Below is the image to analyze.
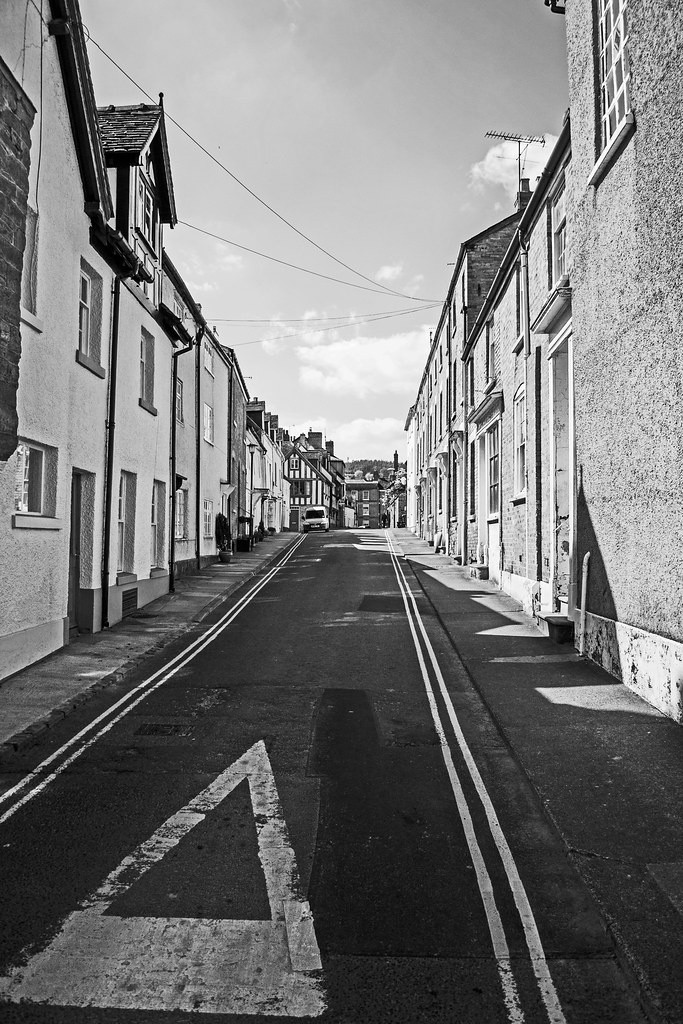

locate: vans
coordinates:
[301,505,330,533]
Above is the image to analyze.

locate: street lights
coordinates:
[247,442,258,539]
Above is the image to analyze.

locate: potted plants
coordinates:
[215,513,234,563]
[268,527,276,536]
